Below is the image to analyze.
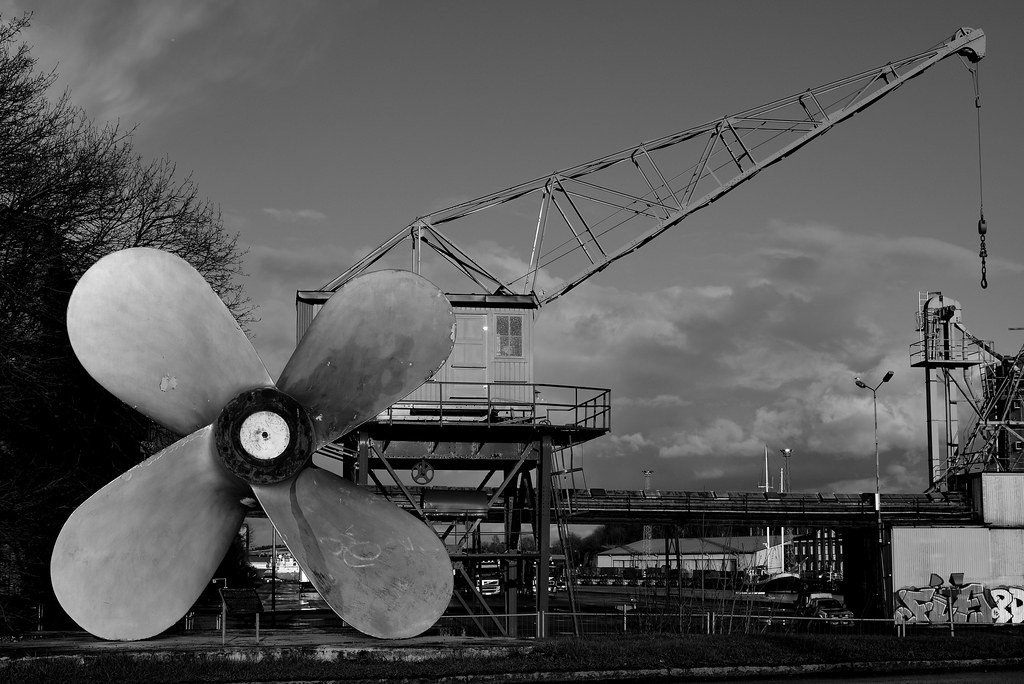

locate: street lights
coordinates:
[780,448,795,574]
[640,468,654,582]
[854,371,894,628]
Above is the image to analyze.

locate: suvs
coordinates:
[809,597,854,634]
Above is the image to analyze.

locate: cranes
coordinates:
[294,27,988,638]
